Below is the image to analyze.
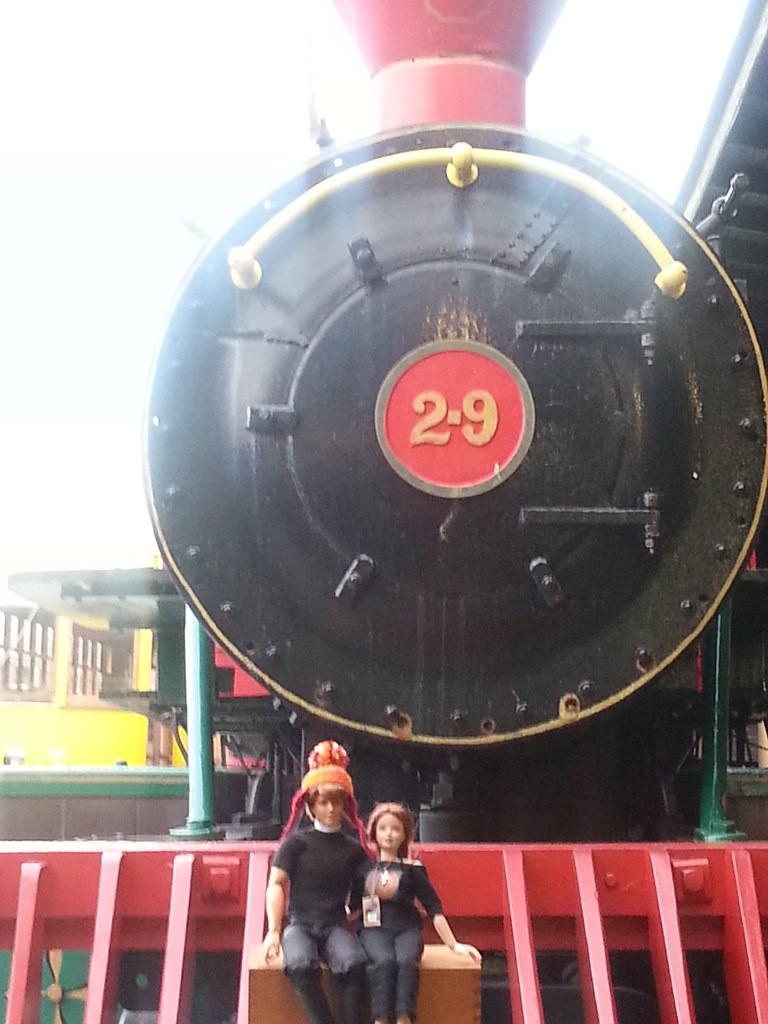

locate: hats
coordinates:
[280,740,376,860]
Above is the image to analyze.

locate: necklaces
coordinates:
[380,861,392,886]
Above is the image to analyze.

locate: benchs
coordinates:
[248,943,482,1024]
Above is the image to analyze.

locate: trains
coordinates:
[140,0,767,987]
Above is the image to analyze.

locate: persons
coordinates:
[347,801,482,1024]
[262,740,371,1024]
